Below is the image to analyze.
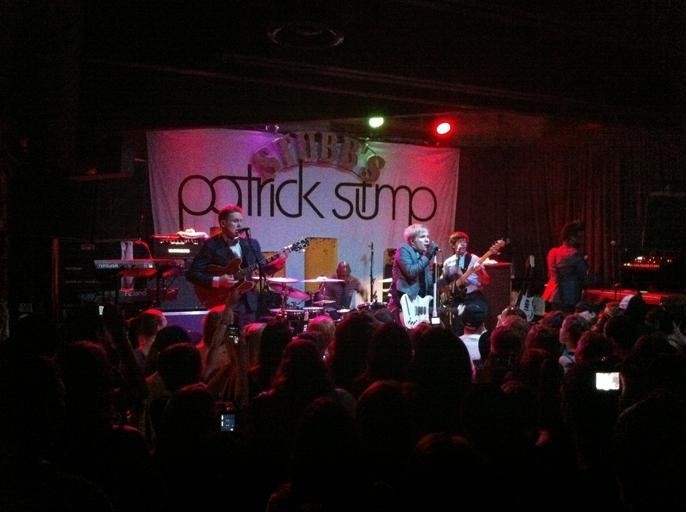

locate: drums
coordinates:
[313,300,338,322]
[302,307,325,330]
[270,308,305,334]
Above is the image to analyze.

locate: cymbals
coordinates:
[303,276,346,283]
[268,285,310,304]
[253,275,301,283]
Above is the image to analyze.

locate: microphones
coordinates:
[238,227,249,233]
[457,244,461,251]
[432,240,441,252]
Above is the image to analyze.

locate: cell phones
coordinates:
[593,369,622,392]
[216,408,238,433]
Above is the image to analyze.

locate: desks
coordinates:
[162,311,209,339]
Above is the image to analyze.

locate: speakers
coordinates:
[483,262,512,331]
[156,262,207,310]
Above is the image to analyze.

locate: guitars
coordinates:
[396,293,466,333]
[192,238,309,313]
[439,239,505,305]
[515,254,535,323]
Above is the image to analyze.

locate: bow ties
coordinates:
[227,239,238,247]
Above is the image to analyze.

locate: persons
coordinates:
[438,231,492,319]
[324,261,367,320]
[0,294,686,511]
[389,223,443,312]
[540,222,611,313]
[185,204,266,329]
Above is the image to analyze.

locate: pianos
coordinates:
[160,240,204,258]
[94,259,188,277]
